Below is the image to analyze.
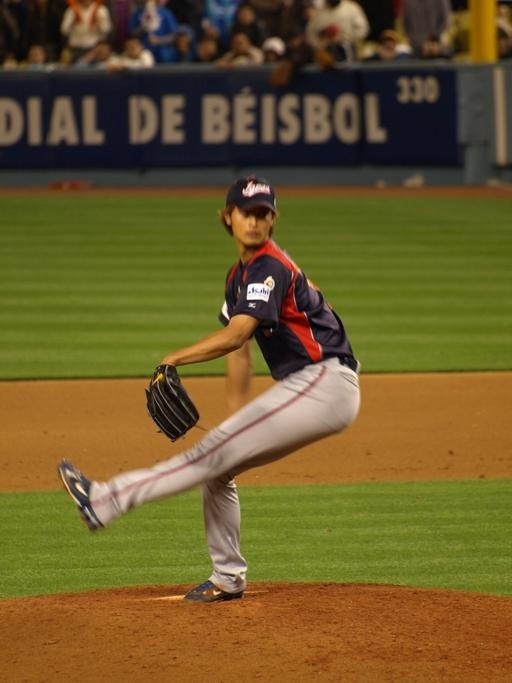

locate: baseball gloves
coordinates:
[147,363,199,441]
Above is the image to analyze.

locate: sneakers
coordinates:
[58,458,101,530]
[185,581,244,603]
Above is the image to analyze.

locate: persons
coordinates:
[56,176,361,601]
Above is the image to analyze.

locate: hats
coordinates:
[318,25,338,40]
[226,177,277,217]
[262,37,285,55]
